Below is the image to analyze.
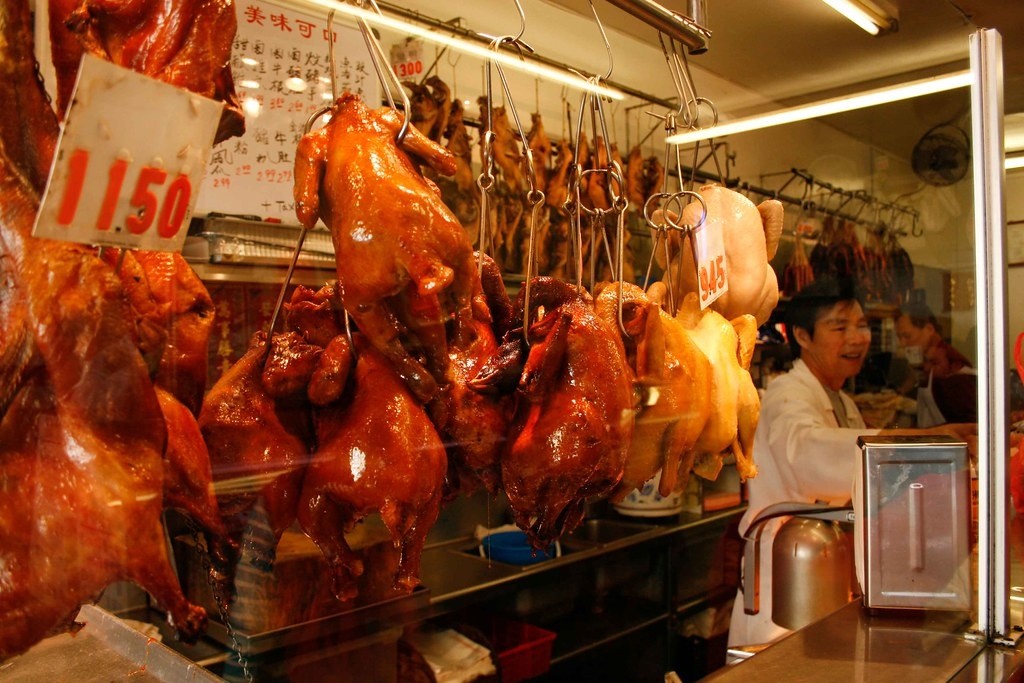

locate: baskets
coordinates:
[464,612,558,683]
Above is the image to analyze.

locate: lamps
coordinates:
[664,69,972,145]
[823,0,898,37]
[289,0,626,103]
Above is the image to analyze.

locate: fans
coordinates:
[910,122,970,187]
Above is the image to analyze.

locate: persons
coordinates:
[924,334,972,381]
[726,280,977,664]
[893,301,978,428]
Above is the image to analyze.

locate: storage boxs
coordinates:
[674,631,729,682]
[398,616,557,683]
[176,532,398,632]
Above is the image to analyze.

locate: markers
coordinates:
[208,211,262,223]
[262,216,300,227]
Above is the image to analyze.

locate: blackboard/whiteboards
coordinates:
[190,0,380,233]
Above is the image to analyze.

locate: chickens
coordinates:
[0,0,916,657]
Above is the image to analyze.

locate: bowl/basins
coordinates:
[481,531,555,565]
